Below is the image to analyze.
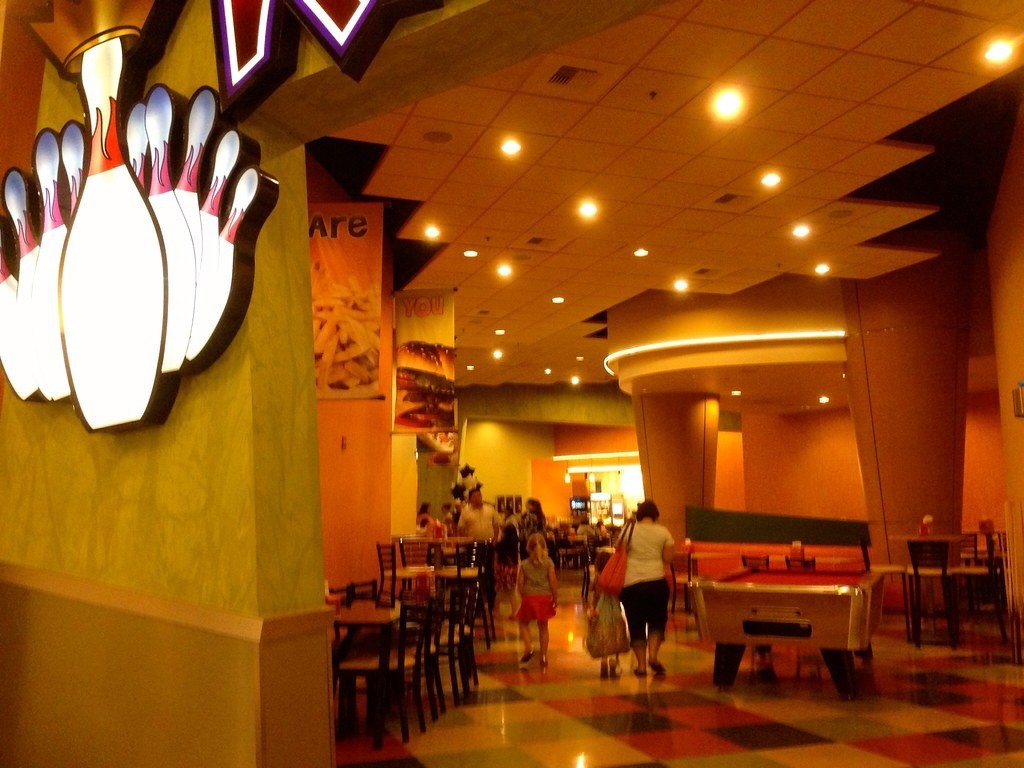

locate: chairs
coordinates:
[904,538,961,647]
[328,536,499,747]
[670,551,701,617]
[969,516,1006,644]
[555,529,610,602]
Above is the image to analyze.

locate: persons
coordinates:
[615,499,674,678]
[516,533,558,667]
[586,551,630,679]
[416,485,614,616]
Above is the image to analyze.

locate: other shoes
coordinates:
[521,646,534,663]
[540,652,548,666]
[610,659,621,679]
[600,661,608,678]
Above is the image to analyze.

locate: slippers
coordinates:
[634,668,647,677]
[649,659,664,672]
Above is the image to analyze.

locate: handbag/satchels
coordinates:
[599,519,635,594]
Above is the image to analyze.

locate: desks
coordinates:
[697,566,883,702]
[330,607,400,725]
[386,565,484,582]
[892,534,976,635]
